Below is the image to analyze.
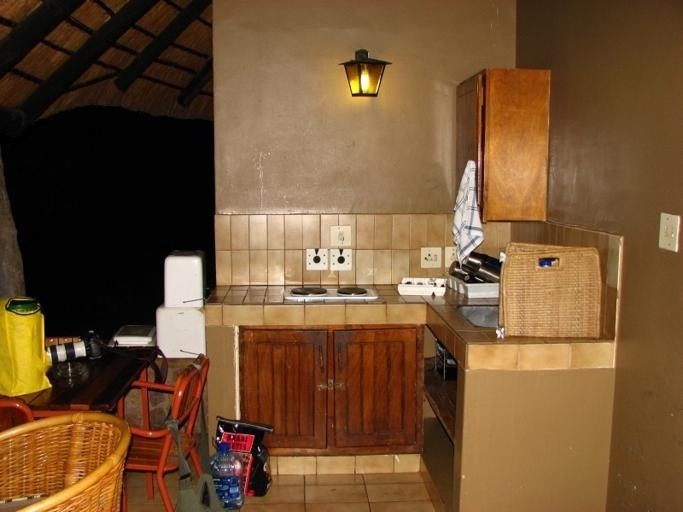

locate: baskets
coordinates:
[497,239,599,338]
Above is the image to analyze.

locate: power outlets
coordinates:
[420,247,441,269]
[445,246,459,269]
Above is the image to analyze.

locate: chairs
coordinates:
[116,353,210,512]
[0,397,36,434]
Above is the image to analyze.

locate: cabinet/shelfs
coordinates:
[420,325,614,512]
[455,67,552,223]
[237,323,425,456]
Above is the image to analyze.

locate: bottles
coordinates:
[211,440,242,508]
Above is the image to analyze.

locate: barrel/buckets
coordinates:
[209,443,244,509]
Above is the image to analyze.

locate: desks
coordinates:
[9,346,161,503]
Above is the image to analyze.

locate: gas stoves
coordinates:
[282,282,374,302]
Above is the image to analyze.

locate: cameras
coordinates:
[46,338,104,361]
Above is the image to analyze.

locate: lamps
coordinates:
[338,48,392,97]
